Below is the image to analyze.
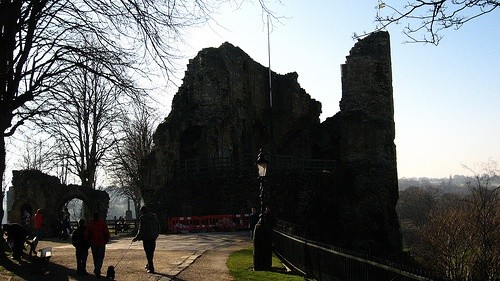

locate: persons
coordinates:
[132,205,160,273]
[249,206,259,239]
[3,224,39,260]
[59,215,73,241]
[71,213,110,277]
[34,209,42,230]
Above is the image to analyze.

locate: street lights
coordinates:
[256,147,269,224]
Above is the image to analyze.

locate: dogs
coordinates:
[105,265,116,281]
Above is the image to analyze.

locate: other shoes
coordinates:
[145,264,154,273]
[76,270,86,277]
